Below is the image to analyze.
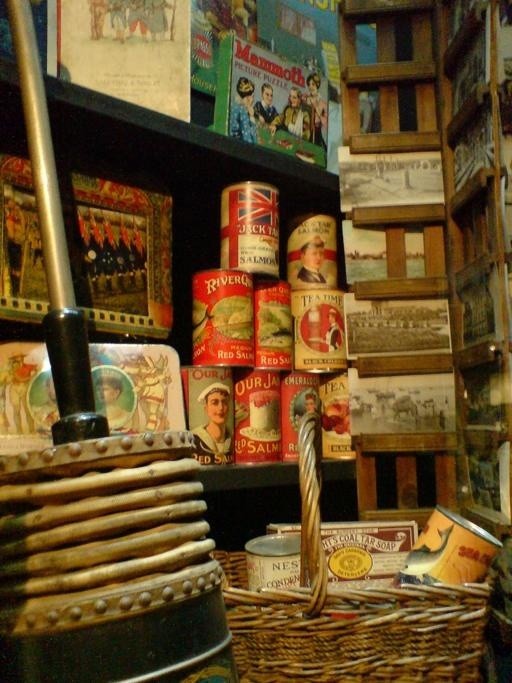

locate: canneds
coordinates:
[234,368,281,466]
[180,365,234,467]
[282,370,322,463]
[191,270,254,367]
[393,504,503,589]
[221,180,280,279]
[254,280,294,370]
[286,212,338,289]
[245,533,302,591]
[322,372,355,460]
[291,289,347,373]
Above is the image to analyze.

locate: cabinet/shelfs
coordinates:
[0,1,357,503]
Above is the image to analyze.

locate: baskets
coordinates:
[209,410,492,682]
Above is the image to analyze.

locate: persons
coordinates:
[32,374,59,424]
[303,392,319,426]
[296,234,327,284]
[90,0,177,46]
[94,368,129,428]
[292,405,307,426]
[5,188,148,299]
[308,308,343,355]
[193,383,233,454]
[204,0,328,146]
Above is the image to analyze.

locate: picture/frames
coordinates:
[0,150,176,339]
